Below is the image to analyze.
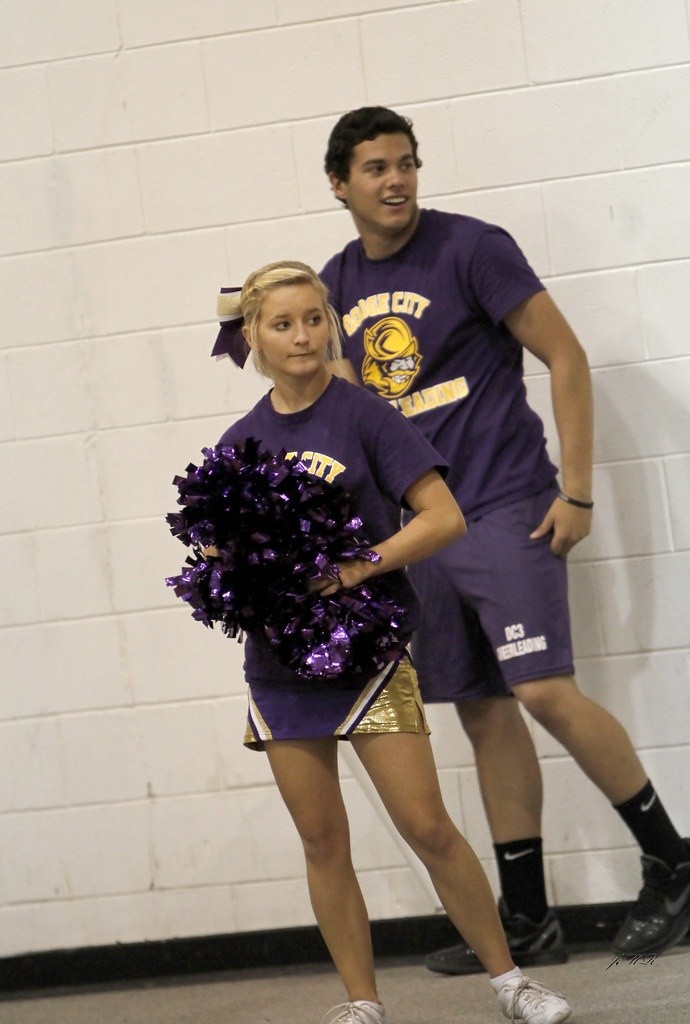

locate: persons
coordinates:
[186,261,576,1024]
[309,105,690,972]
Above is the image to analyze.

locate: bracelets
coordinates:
[557,487,595,512]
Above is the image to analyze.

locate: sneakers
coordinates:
[490,979,573,1024]
[610,835,689,962]
[422,896,571,975]
[327,999,387,1024]
